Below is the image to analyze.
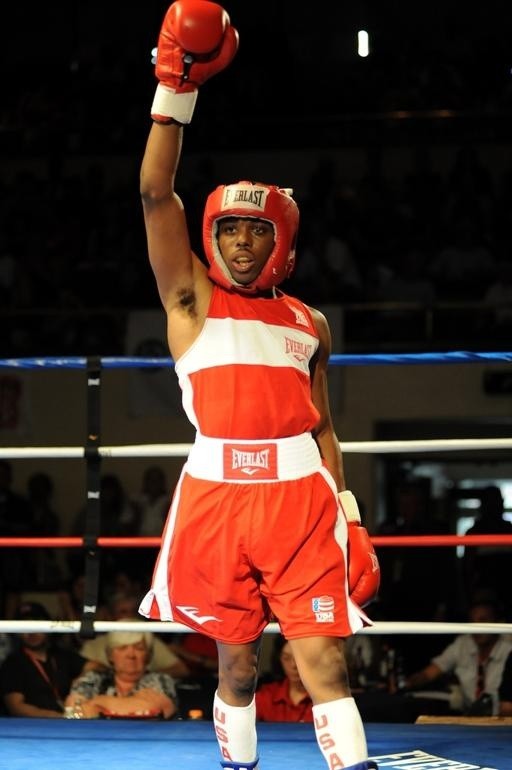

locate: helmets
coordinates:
[201,179,301,296]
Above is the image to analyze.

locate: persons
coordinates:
[137,1,381,770]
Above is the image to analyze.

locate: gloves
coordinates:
[148,0,240,127]
[336,489,381,609]
